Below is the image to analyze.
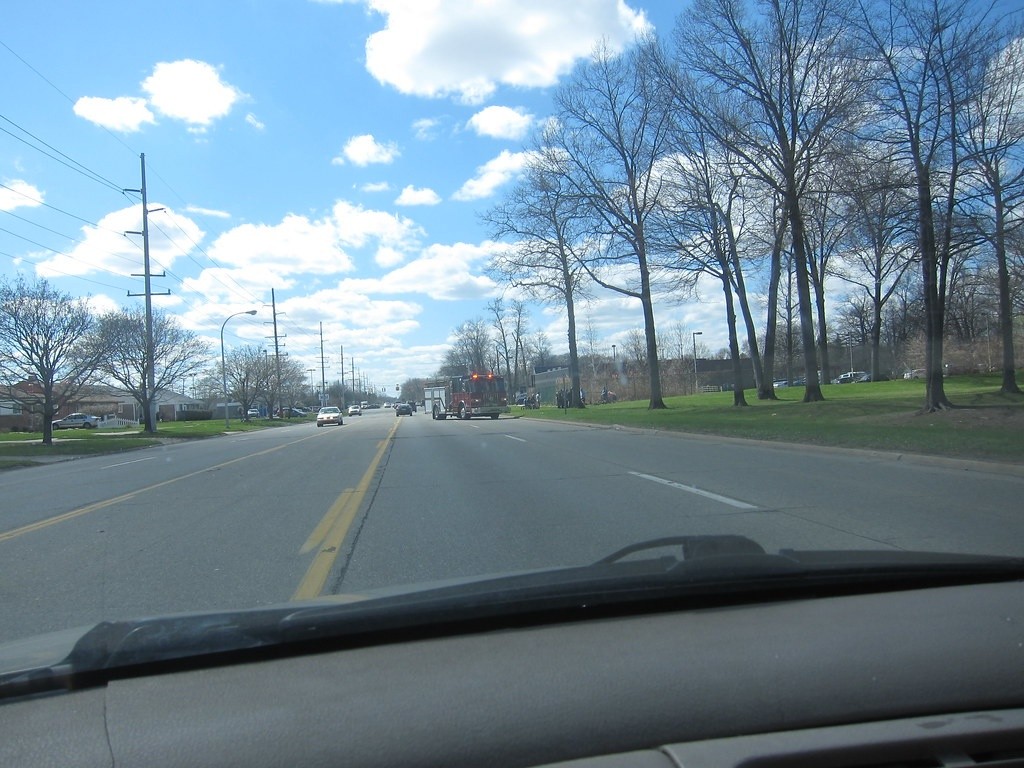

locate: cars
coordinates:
[51,413,101,430]
[396,404,412,417]
[276,407,307,418]
[772,370,888,389]
[247,408,260,418]
[904,368,928,380]
[353,399,425,413]
[317,407,344,427]
[294,405,323,415]
[348,405,362,417]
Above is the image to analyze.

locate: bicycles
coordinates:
[597,393,617,405]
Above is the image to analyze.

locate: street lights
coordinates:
[692,331,703,393]
[981,312,992,373]
[221,309,259,429]
[262,349,269,397]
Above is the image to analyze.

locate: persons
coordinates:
[530,394,536,405]
[601,386,608,404]
[567,386,573,407]
[527,397,530,403]
[580,387,585,404]
[535,392,541,409]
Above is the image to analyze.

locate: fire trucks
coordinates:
[424,373,510,420]
